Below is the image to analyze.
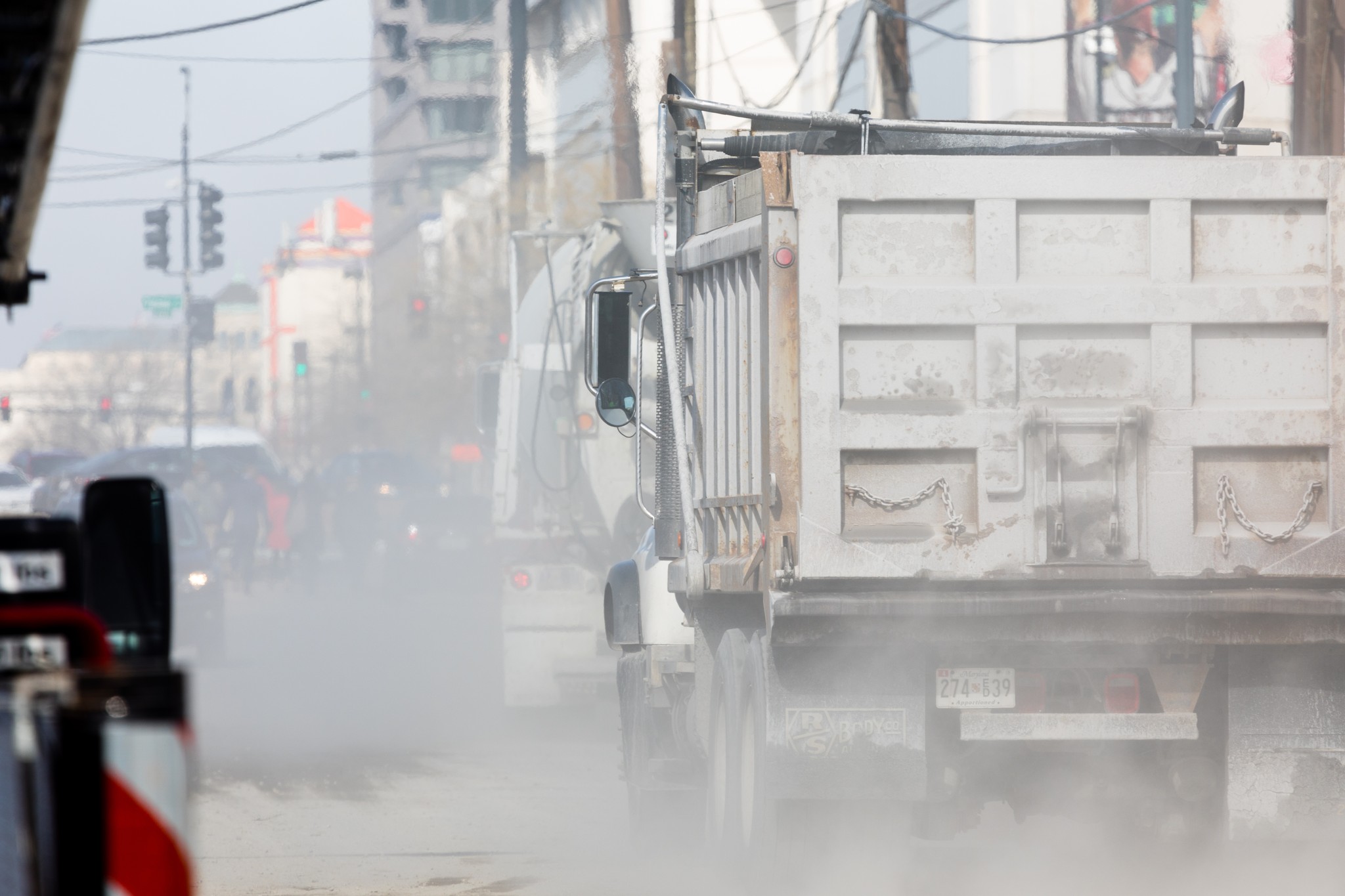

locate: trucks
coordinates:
[584,75,1345,875]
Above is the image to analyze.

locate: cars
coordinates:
[0,423,457,635]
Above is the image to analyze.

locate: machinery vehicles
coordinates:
[480,218,659,728]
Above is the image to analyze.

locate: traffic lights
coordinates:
[360,389,369,399]
[99,395,111,421]
[1,395,10,420]
[198,182,223,270]
[498,331,509,345]
[143,208,168,269]
[412,294,429,347]
[293,340,312,380]
